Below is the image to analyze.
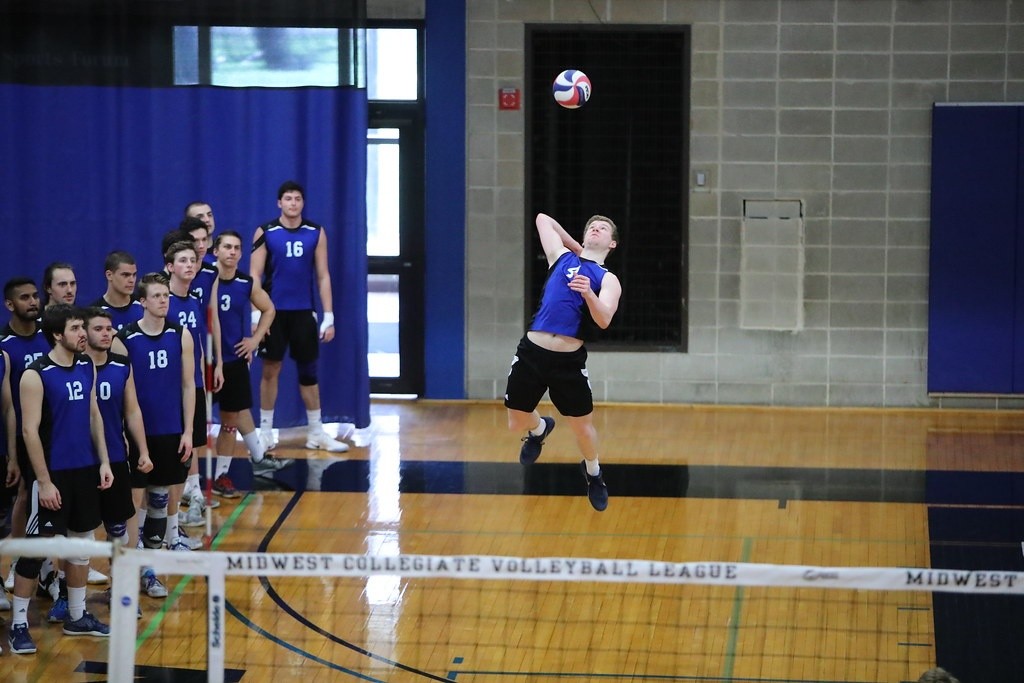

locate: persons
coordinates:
[0,199,282,622]
[6,296,113,654]
[117,273,200,597]
[248,182,350,452]
[504,213,622,512]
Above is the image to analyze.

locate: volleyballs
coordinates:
[553,68,593,111]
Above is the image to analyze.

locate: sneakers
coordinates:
[128,485,223,601]
[62,610,110,636]
[251,456,293,476]
[247,430,277,456]
[36,571,60,602]
[87,567,108,584]
[0,584,10,608]
[519,416,556,465]
[48,599,88,622]
[304,432,346,452]
[211,473,240,499]
[5,563,15,588]
[579,459,609,512]
[8,622,36,653]
